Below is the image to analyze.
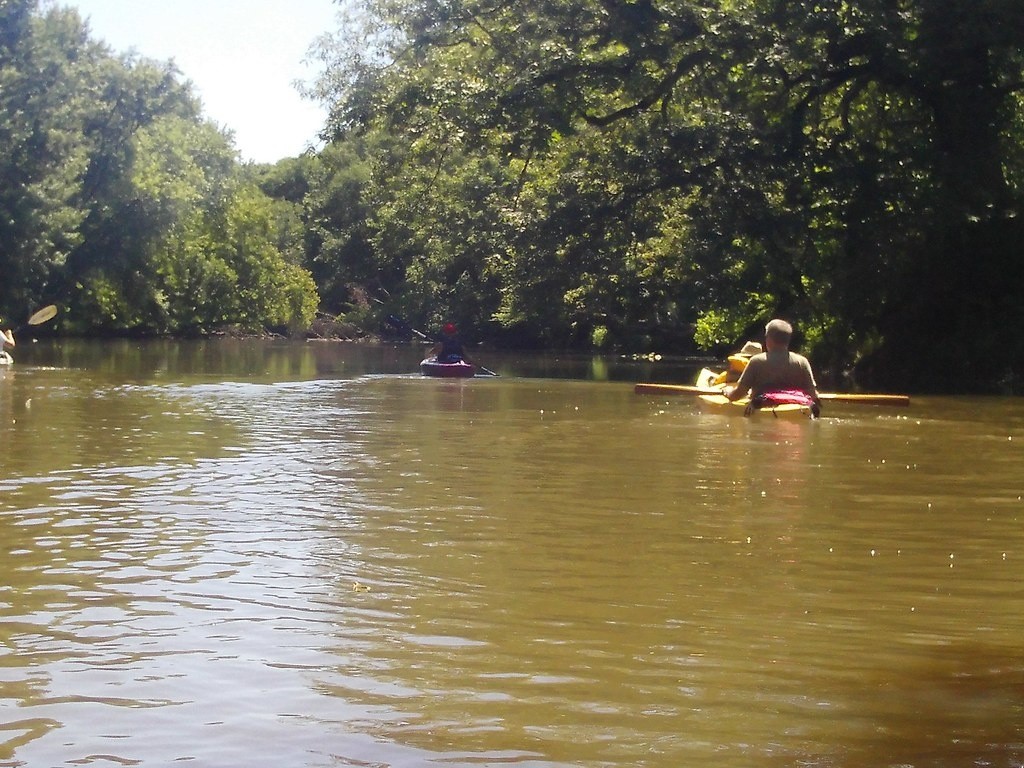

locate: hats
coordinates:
[735,340,762,358]
[442,322,458,335]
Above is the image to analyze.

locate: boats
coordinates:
[0,351,13,368]
[421,356,476,379]
[693,369,815,417]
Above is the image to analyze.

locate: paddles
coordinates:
[632,383,912,409]
[7,303,58,335]
[388,313,498,378]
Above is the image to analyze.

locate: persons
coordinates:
[722,317,822,406]
[0,317,15,351]
[428,323,473,364]
[714,340,763,385]
